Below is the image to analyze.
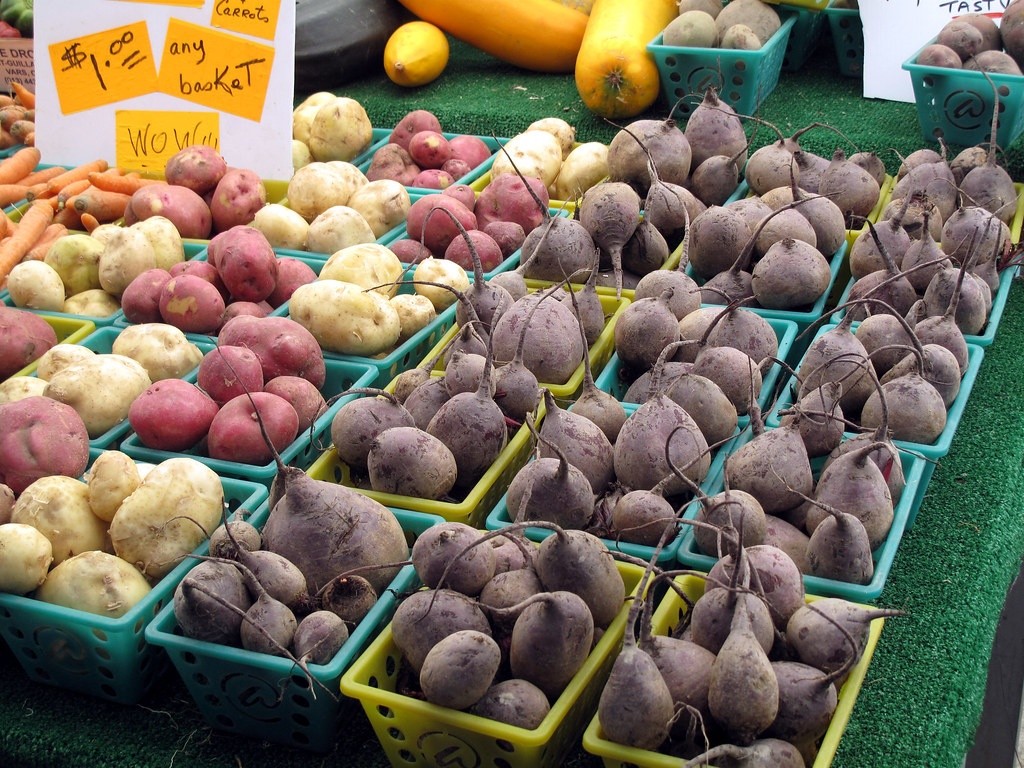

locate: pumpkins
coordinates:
[382,0,677,114]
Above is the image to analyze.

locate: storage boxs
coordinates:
[358,134,512,193]
[830,256,1018,347]
[524,211,684,302]
[0,128,445,735]
[745,173,892,256]
[875,173,1024,253]
[386,208,569,281]
[419,289,630,409]
[583,569,884,768]
[646,11,798,121]
[593,316,799,423]
[679,240,848,324]
[766,325,985,530]
[469,141,612,211]
[485,403,741,563]
[339,529,655,768]
[305,372,547,530]
[902,34,1024,152]
[773,0,864,79]
[678,425,926,603]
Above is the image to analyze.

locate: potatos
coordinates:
[166,90,1023,768]
[916,0,1024,146]
[0,216,609,618]
[126,92,608,271]
[662,0,782,111]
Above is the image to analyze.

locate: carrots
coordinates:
[0,81,168,287]
